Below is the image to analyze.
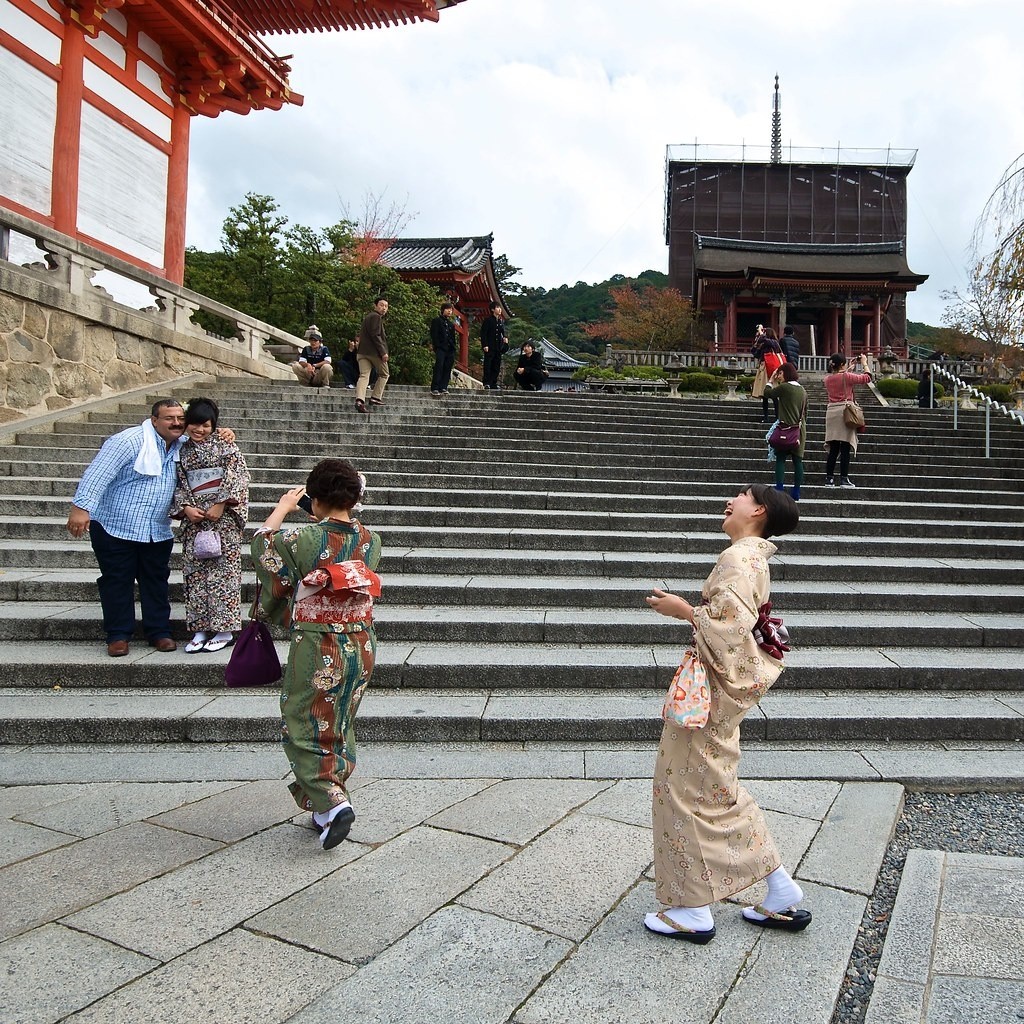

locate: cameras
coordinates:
[856,356,862,364]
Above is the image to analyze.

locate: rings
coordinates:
[70,525,73,529]
[73,527,78,530]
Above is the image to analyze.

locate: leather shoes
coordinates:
[369,397,386,405]
[355,400,369,412]
[156,638,176,652]
[108,639,129,656]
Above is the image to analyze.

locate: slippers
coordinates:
[644,908,716,945]
[741,903,812,931]
[185,631,235,653]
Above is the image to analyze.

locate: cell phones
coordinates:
[296,494,315,517]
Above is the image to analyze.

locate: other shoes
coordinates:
[430,388,449,395]
[346,384,355,389]
[323,386,331,389]
[367,385,371,389]
[497,385,501,388]
[775,483,800,501]
[484,384,490,389]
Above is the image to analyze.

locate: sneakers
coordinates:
[825,474,855,488]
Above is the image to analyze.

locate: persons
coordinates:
[944,354,949,361]
[780,326,799,370]
[250,460,382,849]
[66,399,236,656]
[918,369,938,408]
[824,354,873,488]
[339,334,375,389]
[292,333,333,389]
[751,324,783,423]
[430,303,456,396]
[763,362,809,501]
[354,298,390,412]
[173,396,250,653]
[480,301,509,389]
[940,352,945,363]
[514,340,547,391]
[645,484,811,943]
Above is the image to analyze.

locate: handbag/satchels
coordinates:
[225,574,282,688]
[769,422,801,451]
[193,511,221,559]
[843,403,865,428]
[664,639,712,731]
[764,352,787,380]
[501,343,509,354]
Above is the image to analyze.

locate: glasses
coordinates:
[158,416,186,422]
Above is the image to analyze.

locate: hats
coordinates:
[309,334,321,341]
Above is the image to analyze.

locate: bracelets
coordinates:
[312,364,316,368]
[863,365,867,369]
